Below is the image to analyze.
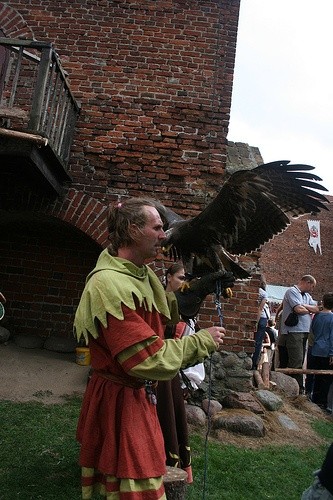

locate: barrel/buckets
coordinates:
[75,347,90,366]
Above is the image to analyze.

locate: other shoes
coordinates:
[302,469,333,500]
[321,403,332,412]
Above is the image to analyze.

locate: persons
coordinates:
[155,261,206,483]
[72,198,236,500]
[257,320,278,371]
[310,292,333,407]
[252,273,270,370]
[276,275,319,393]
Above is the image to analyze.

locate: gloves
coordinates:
[174,271,235,320]
